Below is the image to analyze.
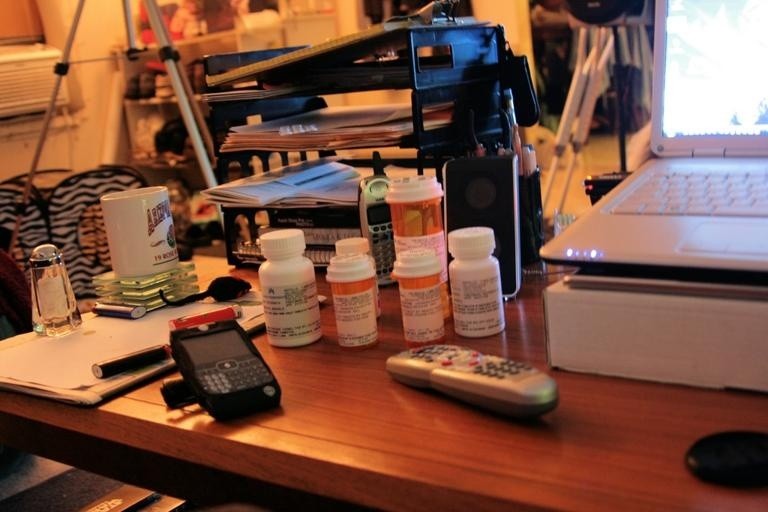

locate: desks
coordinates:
[0,252,768,512]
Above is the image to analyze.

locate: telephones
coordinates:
[358,151,398,286]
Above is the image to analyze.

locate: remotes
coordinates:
[384,342,560,420]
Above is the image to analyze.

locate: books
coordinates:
[1,287,329,407]
[200,14,492,208]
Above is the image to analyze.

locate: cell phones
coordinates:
[168,317,282,422]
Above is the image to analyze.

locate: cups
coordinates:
[99,186,180,278]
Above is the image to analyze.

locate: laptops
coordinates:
[538,0,768,276]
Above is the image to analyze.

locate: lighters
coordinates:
[168,304,244,330]
[90,301,147,319]
[92,344,173,378]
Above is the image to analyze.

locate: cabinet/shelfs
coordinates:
[116,25,301,175]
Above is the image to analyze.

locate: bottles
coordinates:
[384,169,450,322]
[258,229,323,348]
[27,243,83,338]
[388,249,446,348]
[331,252,377,347]
[334,237,381,317]
[448,226,505,337]
